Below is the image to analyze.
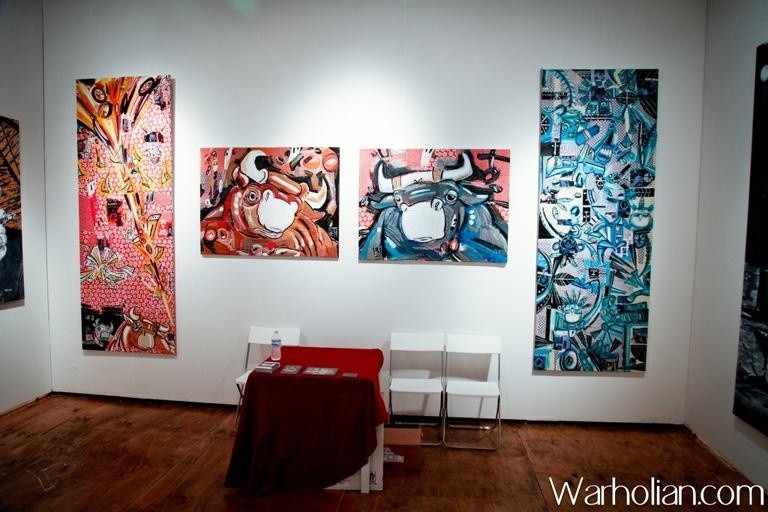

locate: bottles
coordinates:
[271,330,281,361]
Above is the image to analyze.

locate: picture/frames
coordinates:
[357,146,511,266]
[535,67,660,372]
[197,146,344,261]
[732,43,767,435]
[73,74,177,357]
[0,115,24,309]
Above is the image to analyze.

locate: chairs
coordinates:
[386,332,444,448]
[444,332,504,453]
[236,322,294,429]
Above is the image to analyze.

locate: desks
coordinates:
[233,346,387,493]
